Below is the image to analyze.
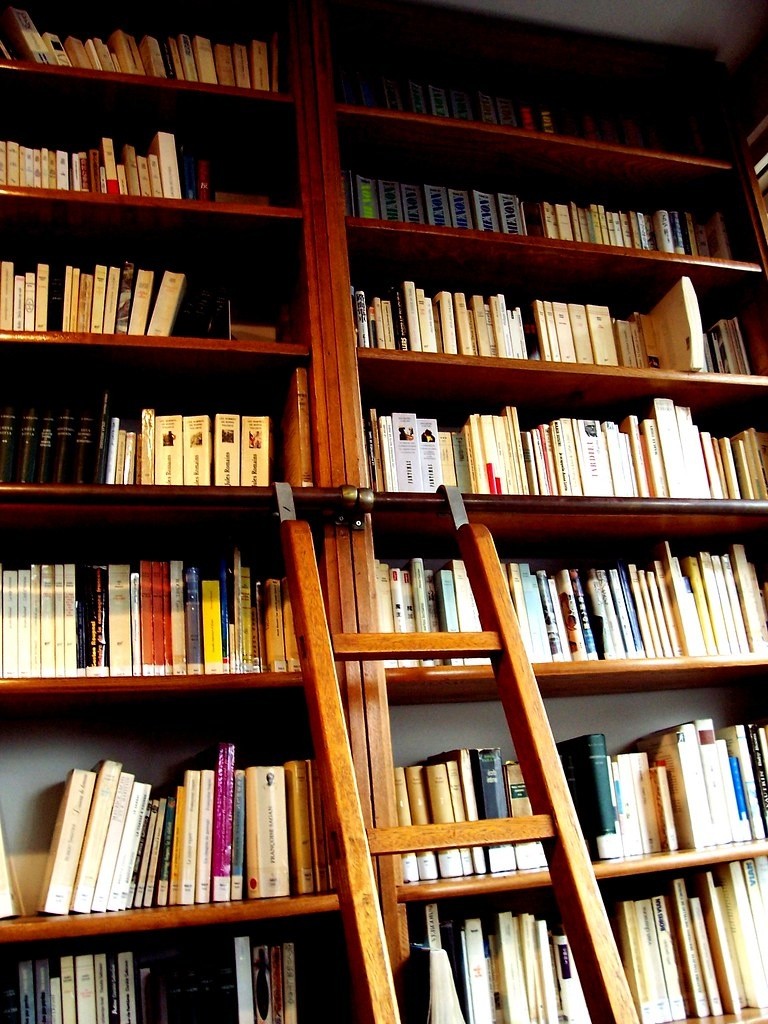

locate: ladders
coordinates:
[273,478,641,1024]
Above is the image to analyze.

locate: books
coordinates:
[329,72,732,162]
[0,367,314,488]
[395,856,768,1024]
[0,6,279,94]
[350,276,751,375]
[0,261,187,337]
[373,541,768,669]
[362,398,768,501]
[0,742,339,920]
[341,167,733,257]
[0,131,210,201]
[0,546,301,679]
[392,717,768,883]
[0,936,298,1024]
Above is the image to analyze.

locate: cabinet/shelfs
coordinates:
[0,0,768,1021]
